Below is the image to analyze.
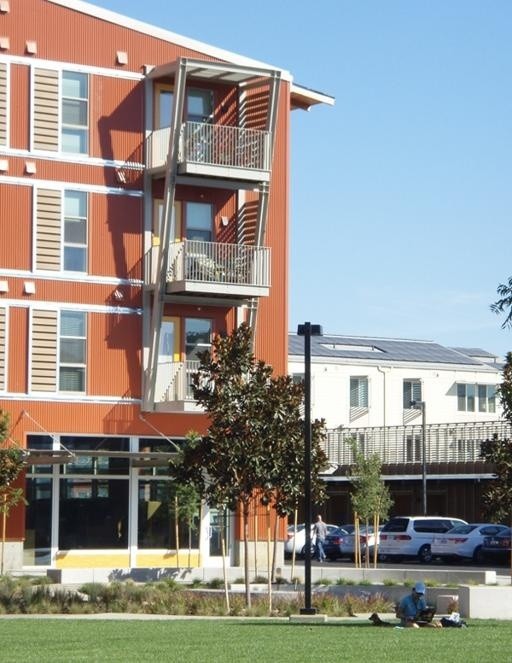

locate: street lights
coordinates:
[289,321,328,621]
[409,399,428,515]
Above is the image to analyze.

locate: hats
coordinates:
[415,582,426,594]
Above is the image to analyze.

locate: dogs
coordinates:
[368,612,394,626]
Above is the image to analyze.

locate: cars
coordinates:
[430,522,509,562]
[482,527,512,565]
[339,524,388,561]
[285,522,347,557]
[324,524,363,562]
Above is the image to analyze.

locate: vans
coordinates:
[379,513,469,563]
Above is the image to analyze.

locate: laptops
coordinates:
[407,608,436,623]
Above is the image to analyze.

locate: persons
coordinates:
[396,580,442,629]
[311,516,327,562]
